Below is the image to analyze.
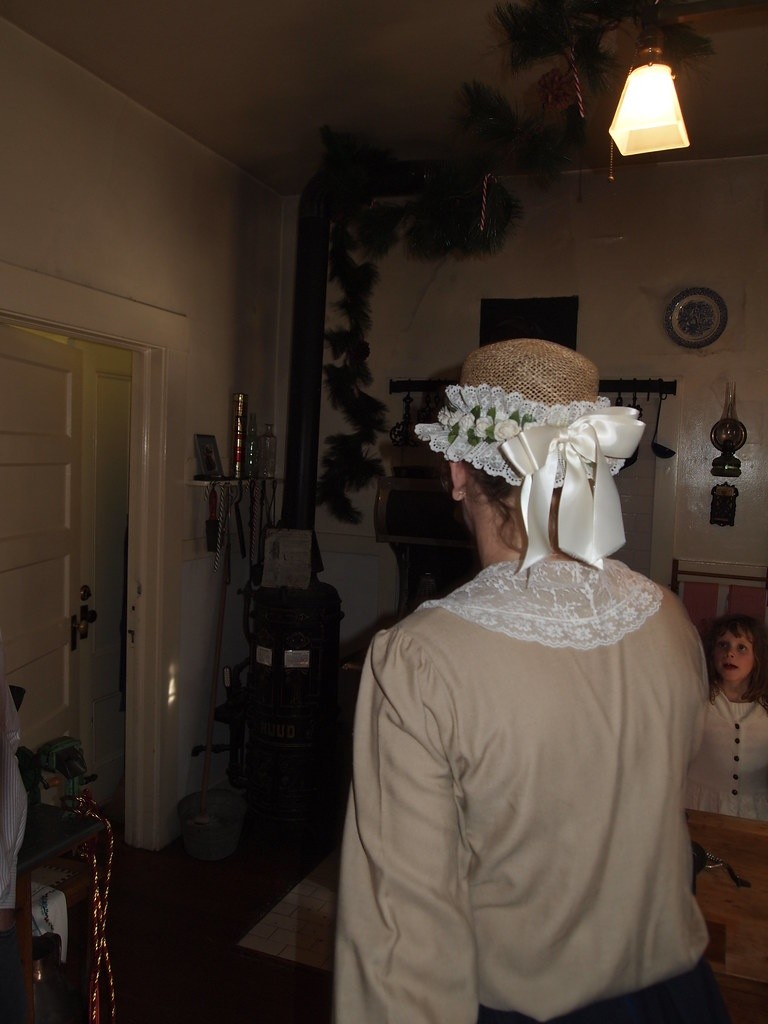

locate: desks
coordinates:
[684,807,768,1024]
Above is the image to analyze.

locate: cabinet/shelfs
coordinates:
[15,803,106,1024]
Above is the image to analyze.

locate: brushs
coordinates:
[205,488,220,554]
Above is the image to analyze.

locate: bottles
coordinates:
[243,414,260,477]
[260,424,277,477]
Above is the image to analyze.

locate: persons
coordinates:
[331,337,743,1024]
[0,684,30,1024]
[698,611,768,822]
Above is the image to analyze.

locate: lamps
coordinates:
[608,0,690,156]
[710,382,747,478]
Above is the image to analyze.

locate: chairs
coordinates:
[671,558,768,648]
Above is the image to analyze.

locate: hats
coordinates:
[414,337,646,576]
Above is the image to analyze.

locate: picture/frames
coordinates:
[194,432,225,477]
